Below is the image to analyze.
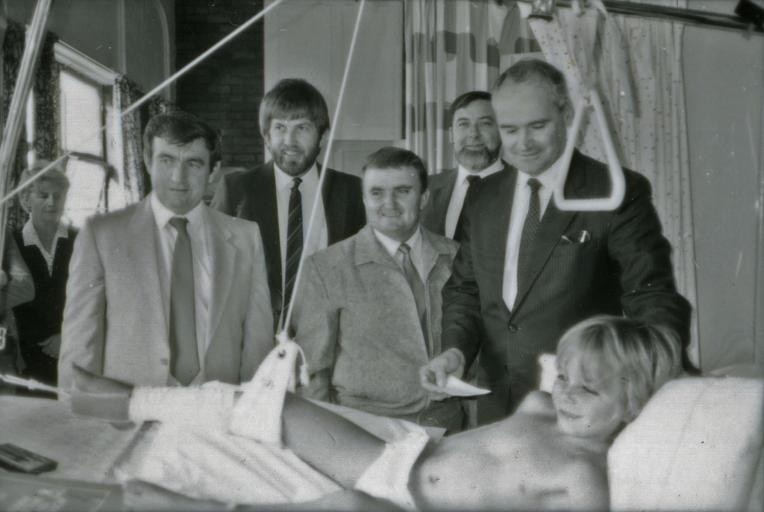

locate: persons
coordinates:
[69,313,683,512]
[419,59,693,429]
[0,229,36,374]
[56,110,276,401]
[209,78,366,342]
[296,146,478,434]
[419,91,509,243]
[10,159,80,398]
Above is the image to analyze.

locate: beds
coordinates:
[0,363,764,512]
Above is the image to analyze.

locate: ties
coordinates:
[517,178,543,288]
[399,243,425,322]
[167,218,200,385]
[450,175,481,239]
[282,178,303,328]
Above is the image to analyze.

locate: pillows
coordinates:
[540,351,764,512]
